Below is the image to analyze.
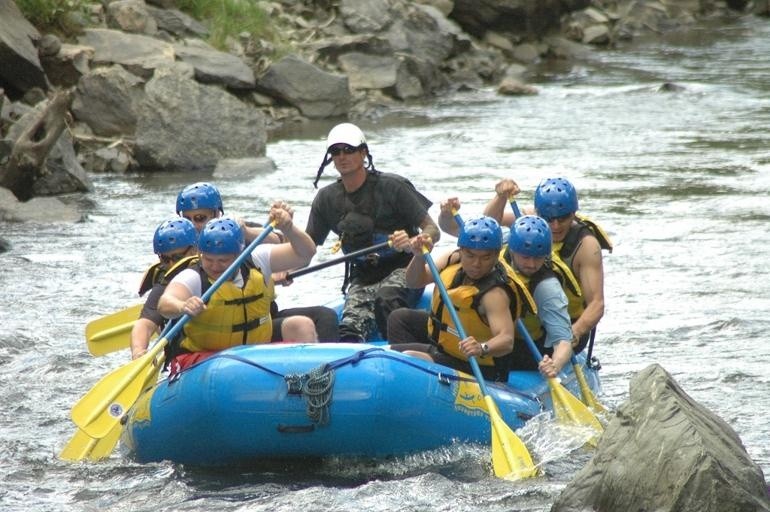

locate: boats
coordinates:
[105,285,608,479]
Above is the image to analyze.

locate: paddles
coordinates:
[62,211,279,465]
[451,206,604,452]
[509,192,613,420]
[85,241,404,358]
[418,242,537,481]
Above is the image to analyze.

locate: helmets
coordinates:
[154,182,245,254]
[327,122,365,149]
[458,177,577,256]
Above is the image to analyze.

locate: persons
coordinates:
[388,215,538,384]
[176,183,338,343]
[438,197,573,378]
[130,200,294,373]
[270,123,441,343]
[158,208,319,373]
[483,178,613,355]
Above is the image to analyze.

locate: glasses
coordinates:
[329,145,357,155]
[161,245,191,263]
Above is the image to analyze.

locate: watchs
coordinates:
[480,343,490,359]
